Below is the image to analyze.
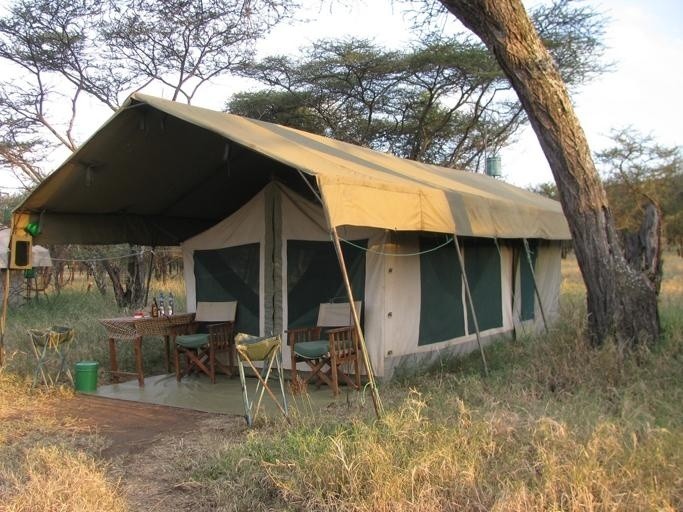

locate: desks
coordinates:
[99,312,196,388]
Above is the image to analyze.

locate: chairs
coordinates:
[284,301,362,397]
[170,301,238,385]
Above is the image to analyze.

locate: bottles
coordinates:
[152,292,175,317]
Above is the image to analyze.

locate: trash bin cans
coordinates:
[76,361,99,392]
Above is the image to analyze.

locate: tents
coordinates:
[9,90,572,385]
